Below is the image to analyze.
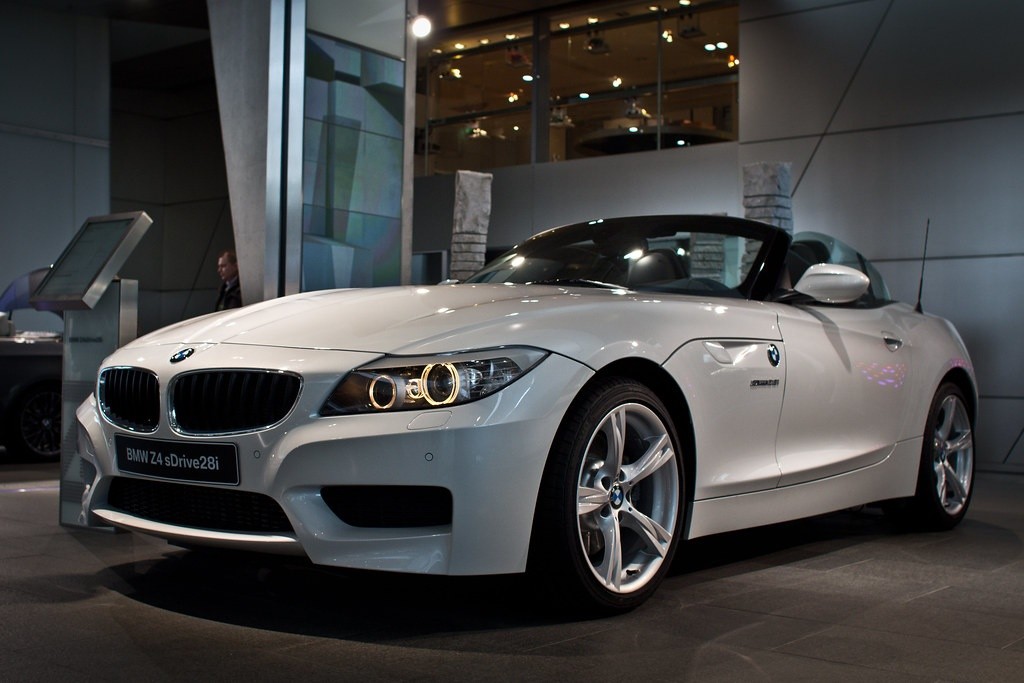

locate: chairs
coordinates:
[632,248,685,283]
[788,239,831,288]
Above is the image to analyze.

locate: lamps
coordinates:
[406,11,431,37]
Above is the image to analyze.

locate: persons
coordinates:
[215,250,242,313]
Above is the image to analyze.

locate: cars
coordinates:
[75,213,981,622]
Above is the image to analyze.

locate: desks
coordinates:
[576,125,737,151]
